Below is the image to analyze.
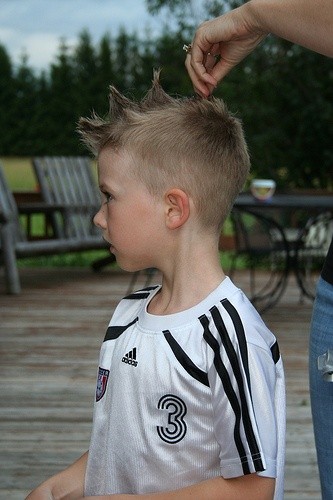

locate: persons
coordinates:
[23,67,288,500]
[185,0,332,500]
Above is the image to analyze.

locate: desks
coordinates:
[229,195,333,315]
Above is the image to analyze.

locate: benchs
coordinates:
[13,156,116,273]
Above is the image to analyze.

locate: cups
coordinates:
[251,179,276,204]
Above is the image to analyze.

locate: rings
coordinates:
[207,51,219,58]
[183,44,191,54]
